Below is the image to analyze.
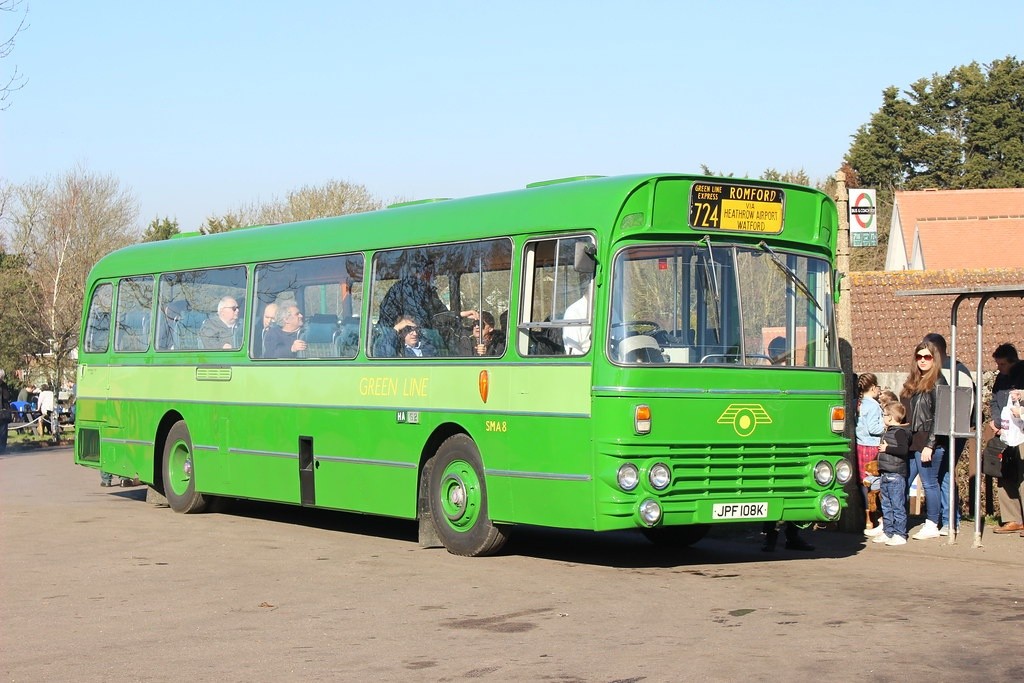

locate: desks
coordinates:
[11,410,43,440]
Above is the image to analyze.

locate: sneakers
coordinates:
[872,532,890,543]
[912,519,940,539]
[939,524,959,536]
[864,519,885,537]
[885,534,906,546]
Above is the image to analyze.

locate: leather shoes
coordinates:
[993,520,1024,533]
[1020,531,1024,537]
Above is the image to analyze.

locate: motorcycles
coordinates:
[10,400,77,424]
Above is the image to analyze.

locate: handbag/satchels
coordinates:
[1000,394,1024,447]
[982,433,1013,478]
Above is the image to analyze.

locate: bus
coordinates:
[0,353,79,392]
[70,173,868,555]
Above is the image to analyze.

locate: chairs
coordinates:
[87,308,723,371]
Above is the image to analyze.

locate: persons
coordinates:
[380,254,448,327]
[257,294,307,358]
[18,383,76,436]
[0,369,13,455]
[858,333,1024,546]
[200,295,240,349]
[564,272,623,356]
[374,311,508,358]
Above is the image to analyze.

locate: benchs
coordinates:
[7,422,38,427]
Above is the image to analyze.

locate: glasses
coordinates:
[915,354,933,360]
[224,306,238,311]
[881,413,889,417]
